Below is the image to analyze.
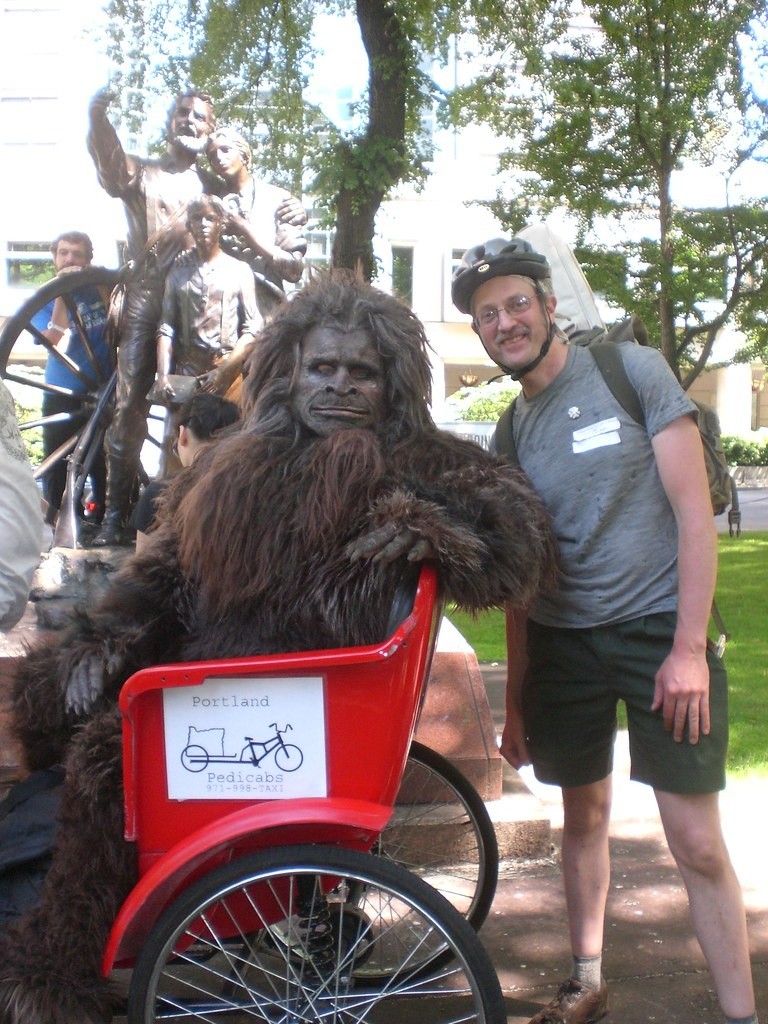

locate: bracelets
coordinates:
[47,321,67,336]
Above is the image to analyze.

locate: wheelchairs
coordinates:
[103,572,508,1024]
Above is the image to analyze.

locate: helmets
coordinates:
[451,238,551,314]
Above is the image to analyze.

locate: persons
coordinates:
[452,233,756,1024]
[27,229,115,527]
[127,393,243,559]
[84,76,311,548]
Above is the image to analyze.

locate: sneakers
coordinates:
[527,977,610,1024]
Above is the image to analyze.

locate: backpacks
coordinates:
[495,316,729,518]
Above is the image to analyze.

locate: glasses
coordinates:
[171,436,179,457]
[473,293,542,329]
